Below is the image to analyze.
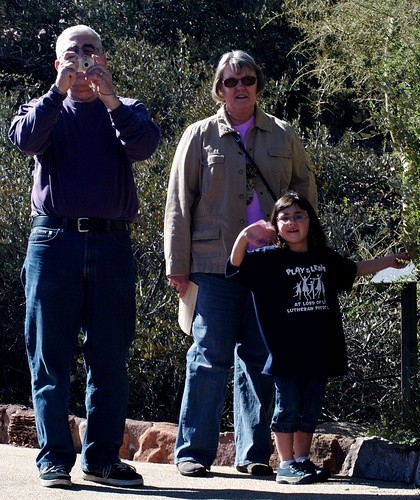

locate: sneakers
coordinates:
[81,461,143,485]
[39,465,71,486]
[276,462,317,484]
[299,459,329,481]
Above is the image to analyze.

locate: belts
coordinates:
[33,215,128,233]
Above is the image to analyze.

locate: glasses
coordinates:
[276,213,308,224]
[222,76,257,88]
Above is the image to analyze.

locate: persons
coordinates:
[7,25,160,487]
[225,192,411,482]
[163,50,317,476]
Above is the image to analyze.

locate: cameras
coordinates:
[75,58,93,71]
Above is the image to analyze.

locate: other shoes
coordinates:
[237,462,273,475]
[177,462,206,476]
